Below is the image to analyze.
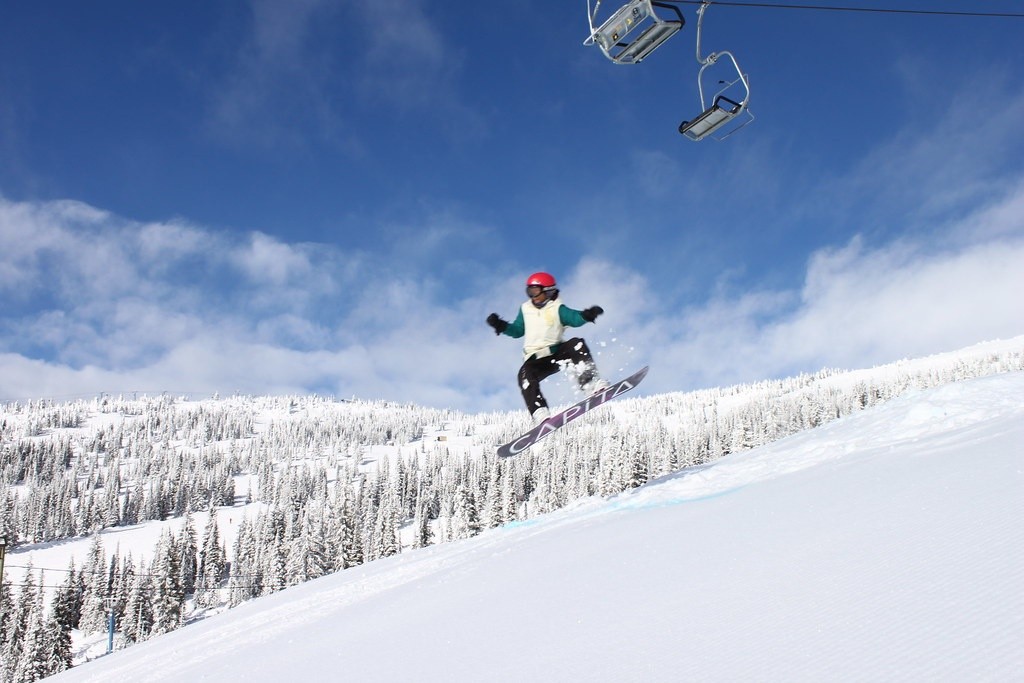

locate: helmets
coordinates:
[526,273,556,309]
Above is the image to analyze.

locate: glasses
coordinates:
[527,286,543,297]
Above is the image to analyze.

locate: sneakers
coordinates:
[532,407,551,426]
[587,379,611,397]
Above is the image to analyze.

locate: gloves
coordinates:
[486,313,509,336]
[582,305,604,324]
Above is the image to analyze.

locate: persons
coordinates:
[485,271,612,428]
